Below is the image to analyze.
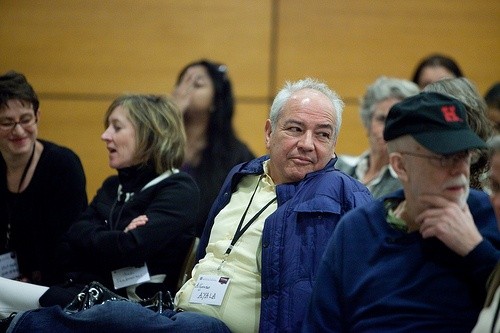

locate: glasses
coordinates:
[0,114,34,131]
[399,150,472,167]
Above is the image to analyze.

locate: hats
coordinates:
[383,90,487,155]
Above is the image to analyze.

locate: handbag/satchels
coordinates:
[67,281,185,317]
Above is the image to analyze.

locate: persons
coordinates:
[305,94,500,333]
[64,92,202,304]
[348,76,422,195]
[481,81,500,135]
[468,134,500,333]
[169,59,258,242]
[0,69,88,284]
[0,78,374,333]
[422,77,494,193]
[409,53,464,91]
